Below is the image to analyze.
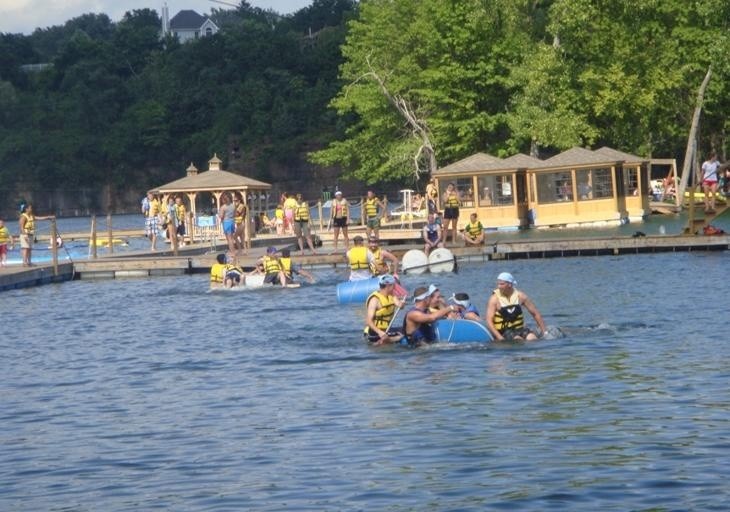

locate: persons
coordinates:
[211,247,312,289]
[345,236,398,280]
[141,193,187,251]
[219,193,247,256]
[363,191,386,244]
[423,183,485,256]
[276,191,318,255]
[367,272,546,346]
[699,152,721,213]
[0,218,13,267]
[331,192,350,254]
[16,194,27,218]
[19,203,55,266]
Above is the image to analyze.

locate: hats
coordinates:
[496,271,519,285]
[378,274,395,285]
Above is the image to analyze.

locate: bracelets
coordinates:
[48,216,50,219]
